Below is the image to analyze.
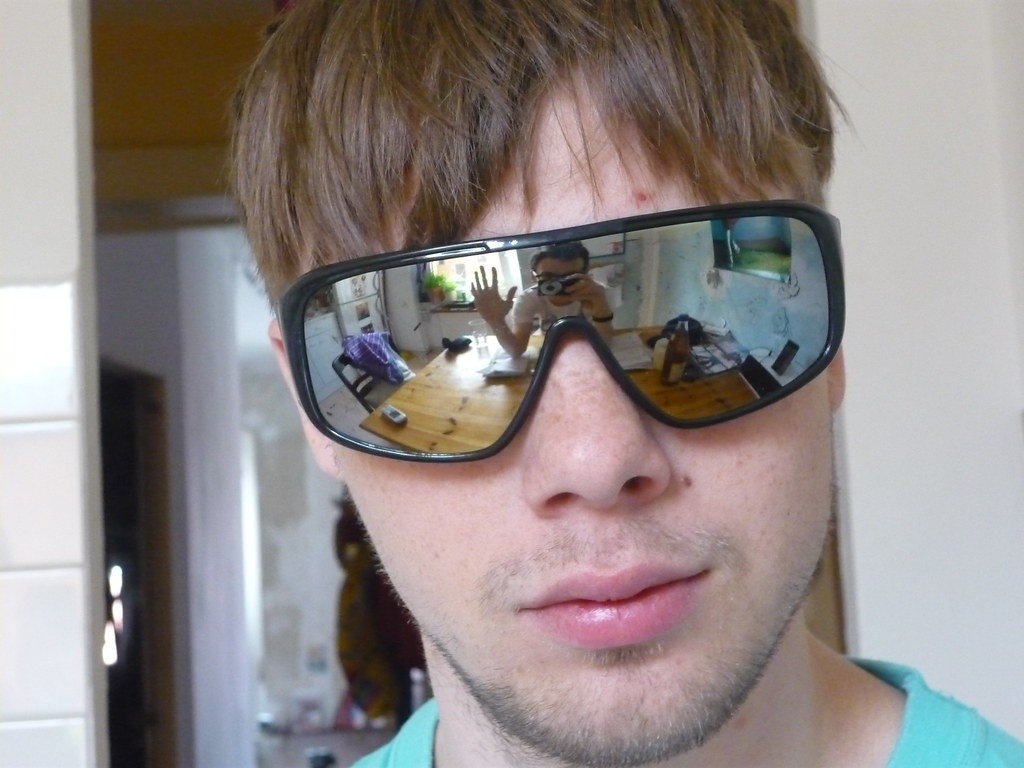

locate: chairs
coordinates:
[332,335,401,414]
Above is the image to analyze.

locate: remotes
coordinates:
[381,405,407,423]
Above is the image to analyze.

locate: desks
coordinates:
[359,325,799,452]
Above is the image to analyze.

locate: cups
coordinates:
[468,319,487,347]
[654,338,668,371]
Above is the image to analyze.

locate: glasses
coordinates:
[529,268,588,280]
[274,199,846,462]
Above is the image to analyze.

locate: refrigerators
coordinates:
[324,271,387,377]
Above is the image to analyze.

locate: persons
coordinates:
[232,0,1023,767]
[471,240,616,357]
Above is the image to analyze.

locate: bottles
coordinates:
[660,314,690,387]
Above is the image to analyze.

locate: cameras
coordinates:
[538,277,577,296]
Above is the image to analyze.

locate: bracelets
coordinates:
[592,313,614,323]
